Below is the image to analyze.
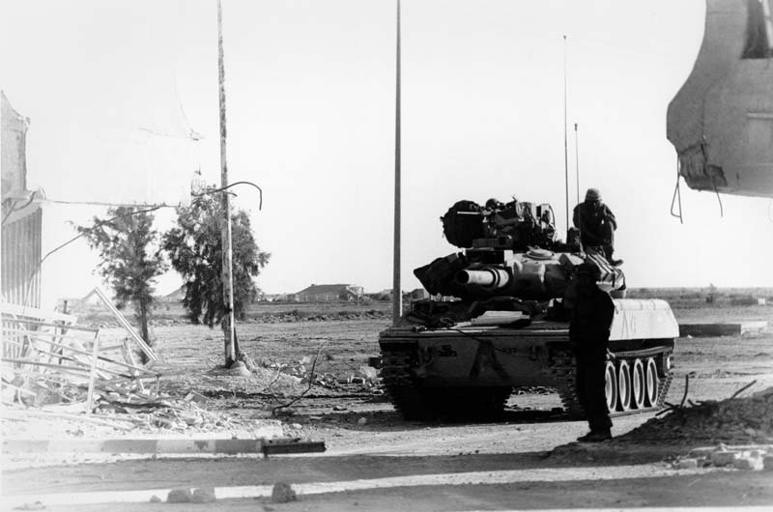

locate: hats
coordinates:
[586,189,600,201]
[579,263,601,280]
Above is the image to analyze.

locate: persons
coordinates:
[558,258,614,444]
[571,189,623,266]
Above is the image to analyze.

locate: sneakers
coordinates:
[611,259,623,265]
[578,431,612,441]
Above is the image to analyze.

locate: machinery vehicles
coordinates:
[378,199,677,413]
